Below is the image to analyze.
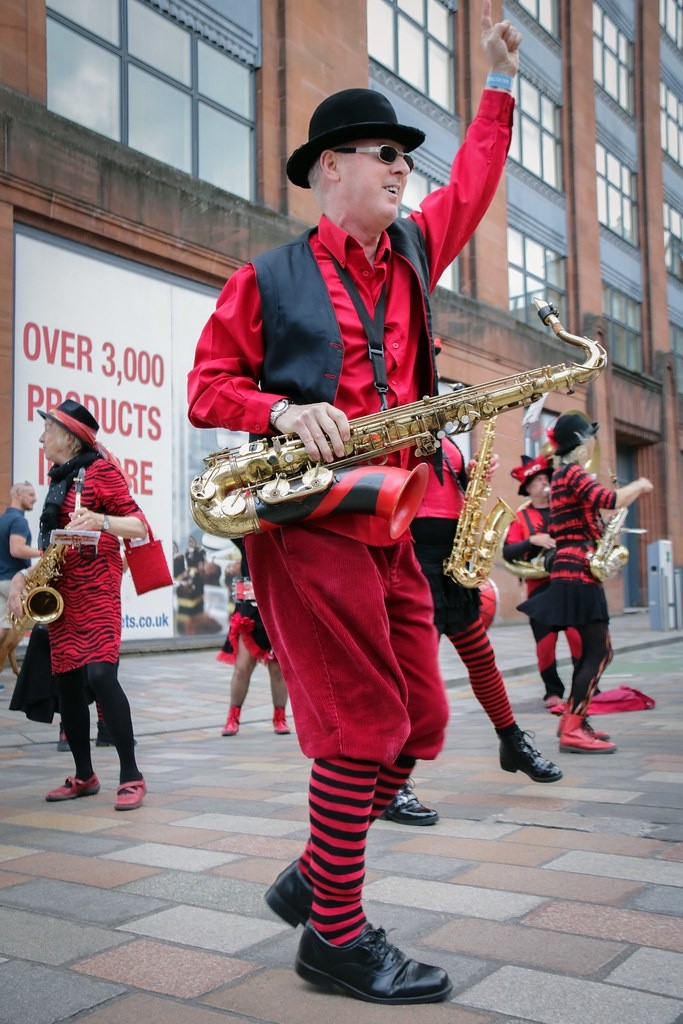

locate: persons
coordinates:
[0,480,44,689]
[216,535,291,737]
[504,455,606,706]
[6,397,149,810]
[517,408,655,752]
[184,19,525,1005]
[377,434,562,829]
[173,534,241,614]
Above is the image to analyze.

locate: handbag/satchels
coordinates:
[123,513,173,596]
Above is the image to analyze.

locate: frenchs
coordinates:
[493,408,604,583]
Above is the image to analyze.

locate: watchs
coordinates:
[102,514,110,532]
[269,398,295,426]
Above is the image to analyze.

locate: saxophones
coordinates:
[192,296,608,542]
[589,467,630,582]
[7,465,85,635]
[443,414,520,588]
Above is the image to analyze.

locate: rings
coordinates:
[85,521,88,524]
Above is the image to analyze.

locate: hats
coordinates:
[510,454,556,497]
[547,409,599,456]
[37,399,100,450]
[285,87,427,189]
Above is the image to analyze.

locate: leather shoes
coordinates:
[263,860,315,929]
[377,776,439,826]
[295,920,453,1004]
[499,730,563,783]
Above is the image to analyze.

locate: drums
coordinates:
[229,575,259,607]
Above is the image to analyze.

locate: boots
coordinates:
[560,714,617,754]
[556,709,610,740]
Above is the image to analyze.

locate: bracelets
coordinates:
[20,568,30,578]
[486,72,514,91]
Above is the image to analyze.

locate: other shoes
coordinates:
[272,706,292,735]
[114,775,147,809]
[544,695,562,708]
[56,722,71,751]
[96,720,137,748]
[45,772,101,801]
[221,706,240,735]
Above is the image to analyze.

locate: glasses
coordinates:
[332,144,414,173]
